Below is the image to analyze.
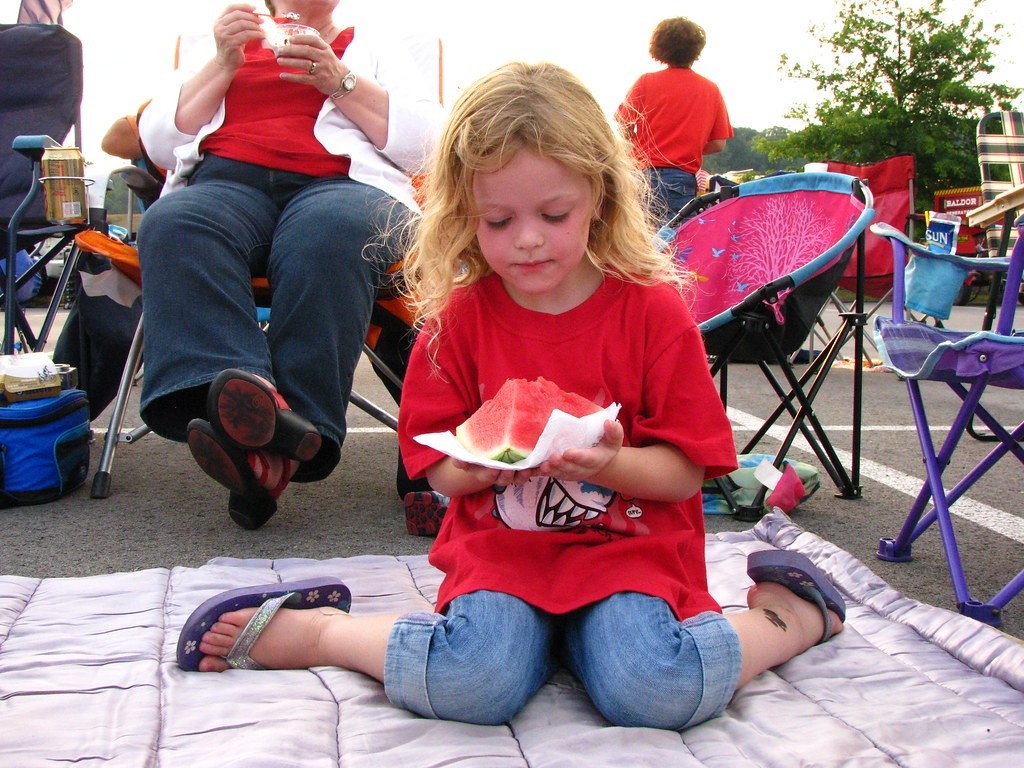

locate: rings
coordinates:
[310,63,316,73]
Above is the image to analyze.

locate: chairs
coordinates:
[651,172,875,523]
[964,110,1024,333]
[809,153,990,376]
[868,213,1024,629]
[0,23,83,356]
[73,98,438,499]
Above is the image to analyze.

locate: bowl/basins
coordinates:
[266,26,319,58]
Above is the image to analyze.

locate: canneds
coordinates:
[41,148,87,225]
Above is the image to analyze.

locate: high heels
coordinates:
[207,369,321,461]
[187,418,289,530]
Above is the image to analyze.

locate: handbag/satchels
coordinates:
[0,388,93,507]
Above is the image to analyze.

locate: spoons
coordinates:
[248,11,296,24]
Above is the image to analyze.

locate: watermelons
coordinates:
[455,376,607,462]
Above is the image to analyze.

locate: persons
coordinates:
[178,63,847,733]
[613,19,732,236]
[137,1,455,530]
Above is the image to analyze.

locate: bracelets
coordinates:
[329,69,358,99]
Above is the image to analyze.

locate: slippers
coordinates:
[747,550,846,646]
[176,576,352,671]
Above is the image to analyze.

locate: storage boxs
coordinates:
[0,352,62,402]
